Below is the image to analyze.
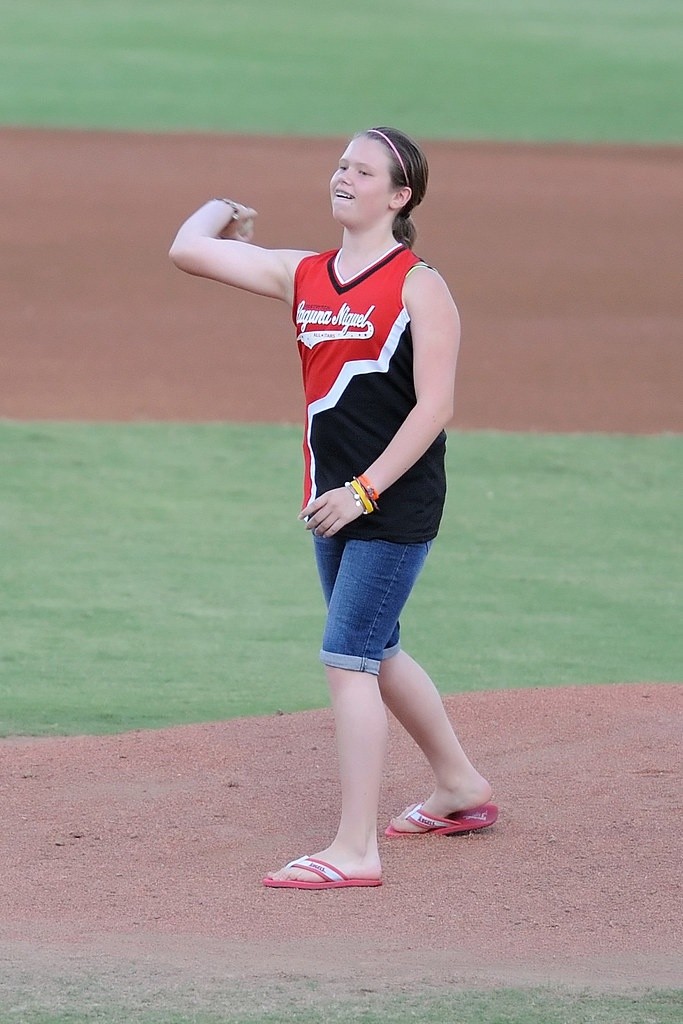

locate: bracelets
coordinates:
[215,197,239,238]
[346,473,381,514]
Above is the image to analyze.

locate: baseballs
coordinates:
[238,217,254,236]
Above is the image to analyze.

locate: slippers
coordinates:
[263,855,383,888]
[383,803,497,836]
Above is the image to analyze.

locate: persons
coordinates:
[165,123,502,892]
